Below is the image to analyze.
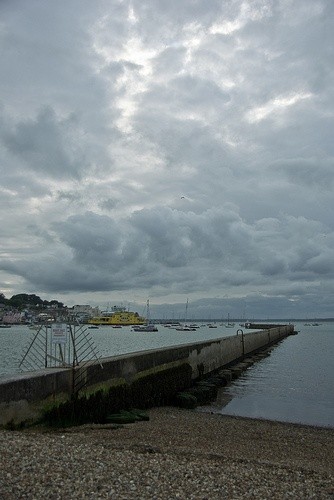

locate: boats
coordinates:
[88,325,99,328]
[131,325,158,331]
[88,306,145,325]
[164,323,244,331]
